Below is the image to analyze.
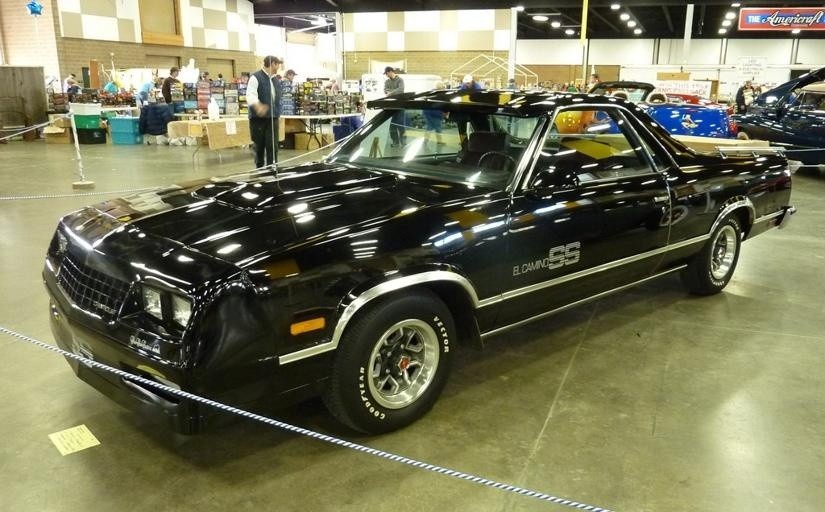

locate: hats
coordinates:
[286,70,298,75]
[383,67,393,74]
[463,75,472,83]
[265,55,283,65]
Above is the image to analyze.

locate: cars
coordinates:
[728,68,825,166]
[666,90,736,135]
[44,90,795,438]
[589,79,730,140]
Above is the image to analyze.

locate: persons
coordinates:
[275,69,298,81]
[736,80,755,112]
[198,71,251,83]
[503,78,522,136]
[383,67,407,146]
[458,76,482,90]
[422,83,452,147]
[329,79,339,91]
[64,74,82,94]
[587,73,609,95]
[245,55,283,166]
[135,67,181,105]
[103,79,120,95]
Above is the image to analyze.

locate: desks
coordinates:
[174,113,249,145]
[278,113,362,147]
[186,116,286,161]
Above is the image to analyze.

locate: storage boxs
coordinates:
[44,103,143,145]
[294,131,323,150]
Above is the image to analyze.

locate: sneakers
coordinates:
[392,137,406,147]
[424,139,447,144]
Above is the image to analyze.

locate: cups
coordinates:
[197,112,202,121]
[189,114,195,121]
